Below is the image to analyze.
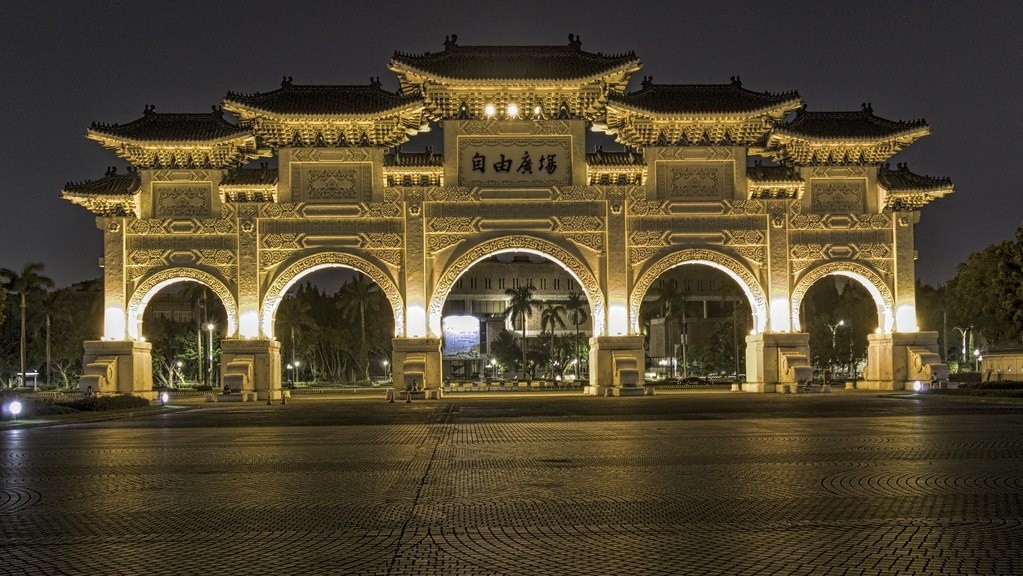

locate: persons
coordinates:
[526,360,533,386]
[931,372,939,390]
[410,379,419,392]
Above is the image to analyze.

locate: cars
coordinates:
[681,376,713,385]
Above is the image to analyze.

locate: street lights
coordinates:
[974,349,980,373]
[208,323,216,388]
[295,361,299,382]
[383,360,389,381]
[491,358,496,380]
[962,325,975,364]
[832,319,845,379]
